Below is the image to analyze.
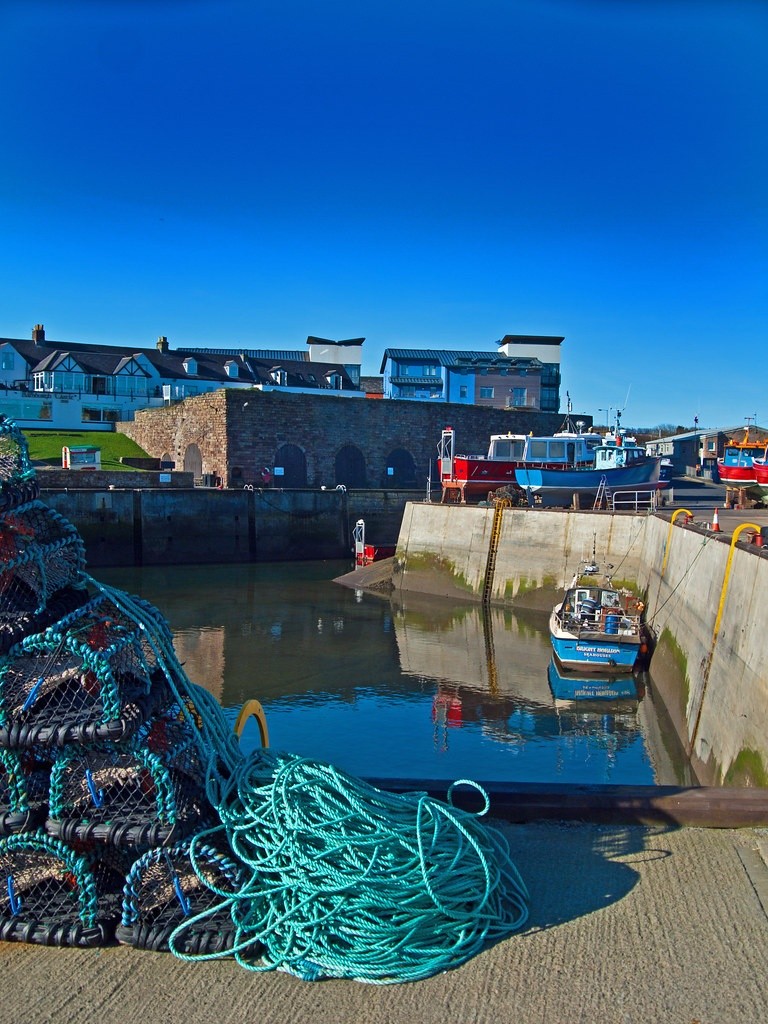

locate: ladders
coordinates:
[593,474,616,510]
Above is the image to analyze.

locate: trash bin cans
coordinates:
[201,473,214,487]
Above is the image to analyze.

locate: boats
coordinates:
[715,414,768,507]
[513,384,661,509]
[548,529,650,674]
[437,389,605,498]
